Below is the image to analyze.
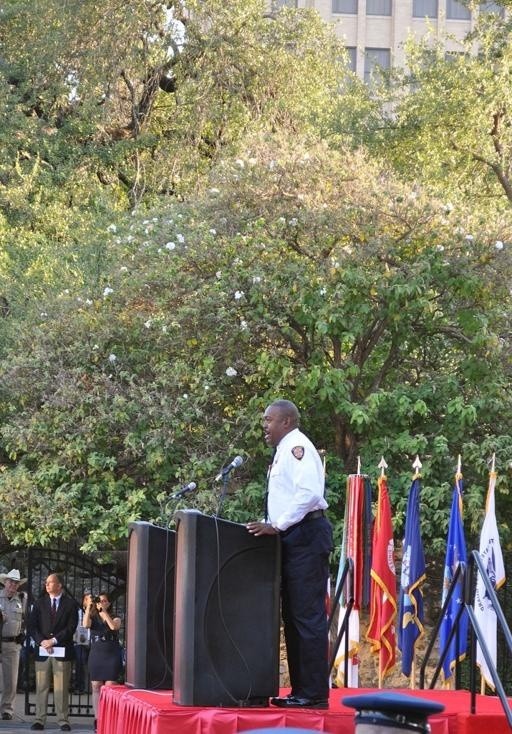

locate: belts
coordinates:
[302,509,325,522]
[1,636,18,642]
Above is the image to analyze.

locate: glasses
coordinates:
[101,599,109,604]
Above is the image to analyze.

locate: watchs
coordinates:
[271,522,280,533]
[50,638,55,646]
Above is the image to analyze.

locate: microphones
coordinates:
[169,481,196,499]
[215,456,243,482]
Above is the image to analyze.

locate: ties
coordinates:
[51,599,57,624]
[265,447,276,521]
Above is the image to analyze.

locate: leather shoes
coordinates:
[31,723,44,730]
[271,692,328,709]
[61,724,71,731]
[2,712,12,720]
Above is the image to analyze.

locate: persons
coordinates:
[28,573,78,731]
[82,594,123,732]
[0,569,28,720]
[73,594,92,695]
[246,400,334,710]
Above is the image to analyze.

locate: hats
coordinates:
[0,569,28,586]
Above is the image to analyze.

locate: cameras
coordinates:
[91,595,100,603]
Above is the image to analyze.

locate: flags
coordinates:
[397,473,428,687]
[440,473,469,688]
[324,576,333,690]
[472,471,507,692]
[333,606,360,689]
[365,477,398,682]
